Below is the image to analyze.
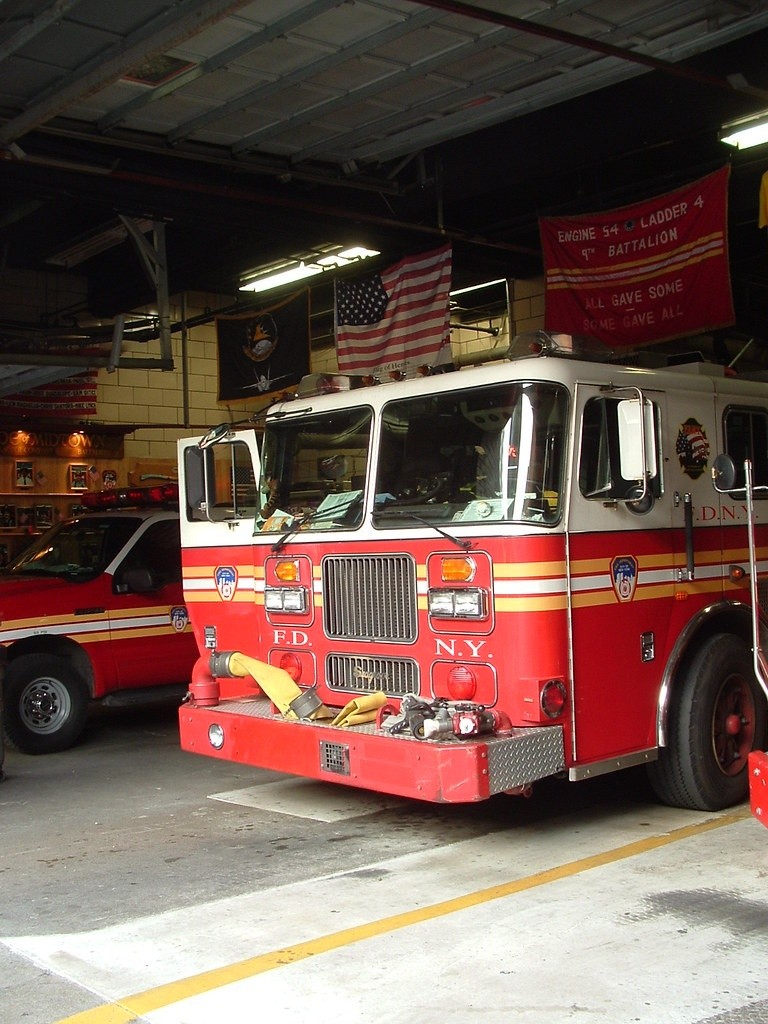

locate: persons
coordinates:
[2,460,89,527]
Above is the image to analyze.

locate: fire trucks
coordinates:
[176,351,768,810]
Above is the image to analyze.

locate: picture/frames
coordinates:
[18,507,34,528]
[0,504,17,529]
[69,503,88,517]
[14,459,35,488]
[35,506,55,528]
[69,463,89,490]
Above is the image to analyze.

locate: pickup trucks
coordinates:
[0,481,200,756]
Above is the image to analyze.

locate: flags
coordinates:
[334,244,453,374]
[214,284,312,406]
[537,163,735,350]
